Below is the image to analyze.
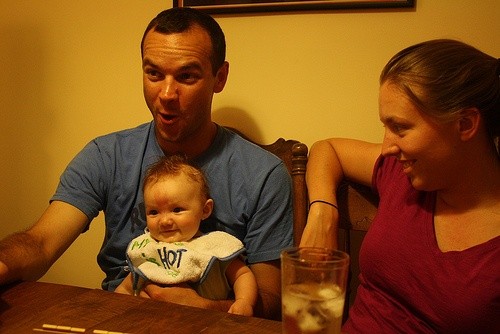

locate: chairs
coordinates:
[223,126,379,326]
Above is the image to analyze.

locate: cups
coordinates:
[280,246,350,334]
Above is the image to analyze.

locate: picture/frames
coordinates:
[173,0,417,16]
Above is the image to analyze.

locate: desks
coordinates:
[0,280,283,334]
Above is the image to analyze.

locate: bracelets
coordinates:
[309,200,339,212]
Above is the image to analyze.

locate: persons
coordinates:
[0,7,294,320]
[114,155,257,317]
[300,38,500,334]
